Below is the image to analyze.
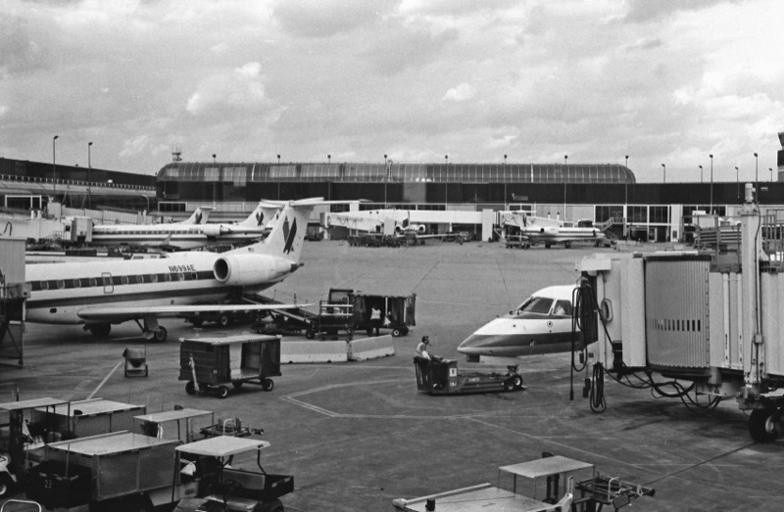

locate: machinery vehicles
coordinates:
[0,392,295,512]
[393,447,655,511]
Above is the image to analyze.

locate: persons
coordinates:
[368,303,382,336]
[416,335,429,384]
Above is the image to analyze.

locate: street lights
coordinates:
[503,154,507,214]
[710,154,714,216]
[383,155,388,209]
[88,141,93,210]
[52,135,59,205]
[563,155,567,222]
[625,154,629,206]
[445,155,448,210]
[735,167,740,205]
[753,153,759,205]
[662,163,666,183]
[212,154,218,207]
[699,165,703,185]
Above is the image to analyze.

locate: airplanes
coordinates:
[455,286,587,366]
[327,156,331,204]
[27,198,344,344]
[63,193,320,262]
[322,197,463,246]
[500,209,618,252]
[276,155,280,202]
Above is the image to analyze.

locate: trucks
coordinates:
[588,186,784,444]
[309,287,417,340]
